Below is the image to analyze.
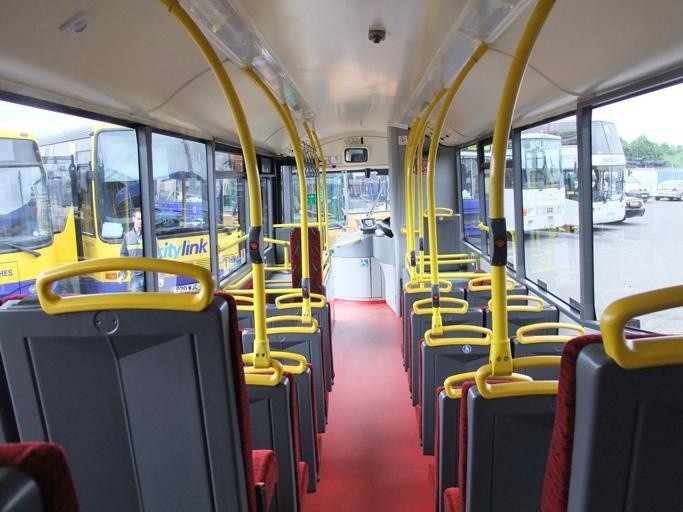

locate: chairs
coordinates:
[435,356,562,512]
[423,212,461,272]
[542,284,683,511]
[402,273,585,456]
[235,292,335,512]
[0,258,279,511]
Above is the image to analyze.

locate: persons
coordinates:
[117,208,162,291]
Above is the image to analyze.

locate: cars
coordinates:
[625,176,683,217]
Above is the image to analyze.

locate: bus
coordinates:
[0,131,61,302]
